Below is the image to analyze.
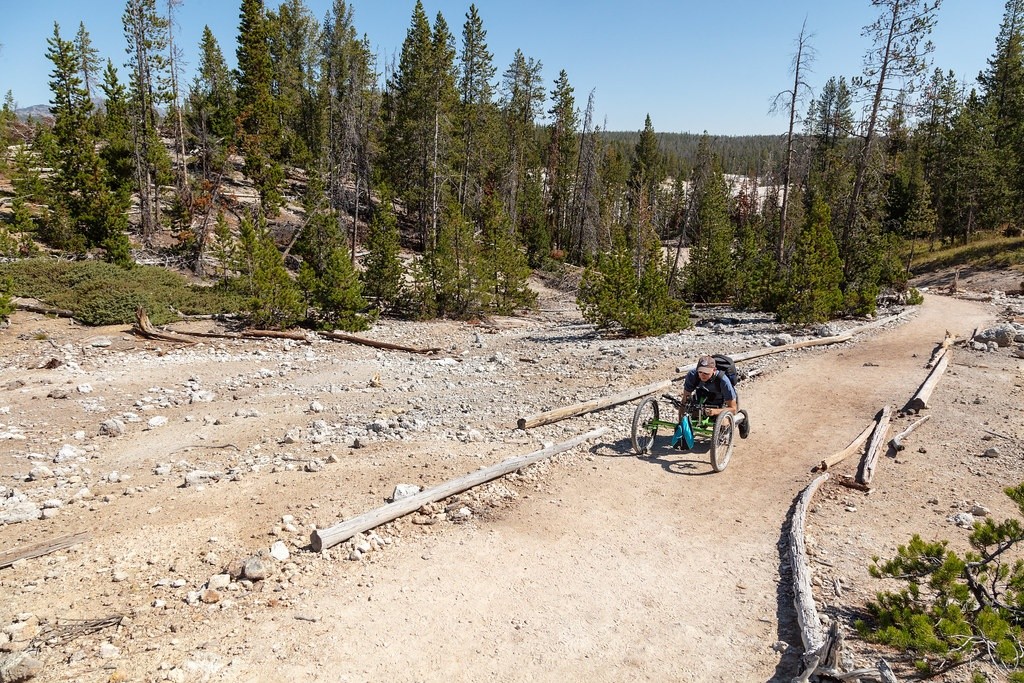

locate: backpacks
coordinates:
[710,354,737,392]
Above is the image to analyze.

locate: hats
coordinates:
[695,355,716,373]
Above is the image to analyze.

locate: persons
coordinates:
[679,355,750,447]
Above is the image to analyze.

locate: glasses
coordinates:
[699,371,709,376]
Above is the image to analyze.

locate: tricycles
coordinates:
[631,394,739,473]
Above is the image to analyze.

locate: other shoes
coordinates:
[738,410,750,439]
